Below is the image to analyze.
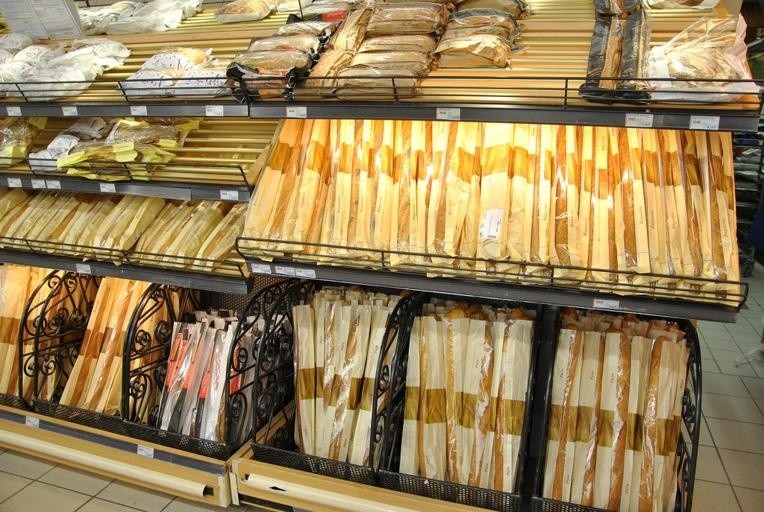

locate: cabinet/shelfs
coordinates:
[1,116,279,510]
[0,0,764,133]
[230,120,750,511]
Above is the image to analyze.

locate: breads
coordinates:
[0,264,93,413]
[238,119,741,304]
[228,20,336,105]
[398,295,534,508]
[214,0,269,24]
[157,307,288,451]
[0,33,122,100]
[0,117,44,168]
[29,115,203,180]
[78,0,198,34]
[122,45,231,98]
[53,278,197,440]
[644,37,745,103]
[543,306,691,512]
[0,191,249,272]
[297,0,527,103]
[294,287,415,483]
